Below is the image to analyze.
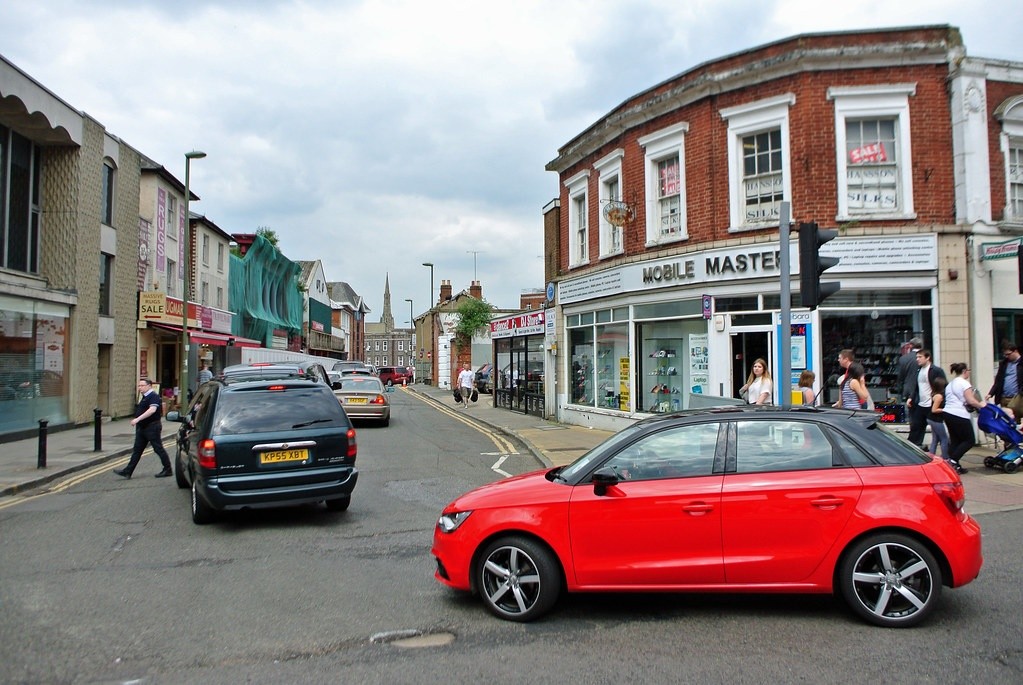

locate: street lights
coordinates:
[181,151,207,416]
[404,299,412,382]
[466,251,485,280]
[422,263,434,387]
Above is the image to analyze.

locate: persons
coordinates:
[1000,406,1023,430]
[831,348,875,411]
[113,378,173,478]
[739,358,773,405]
[457,363,476,409]
[197,363,213,385]
[795,370,815,405]
[896,337,949,460]
[178,400,203,454]
[984,340,1023,449]
[943,362,987,475]
[507,364,517,403]
[408,366,414,383]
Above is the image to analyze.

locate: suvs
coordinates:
[499,360,543,389]
[474,363,493,394]
[166,362,359,524]
[377,366,410,387]
[1,370,63,397]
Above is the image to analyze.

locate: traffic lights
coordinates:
[799,222,839,313]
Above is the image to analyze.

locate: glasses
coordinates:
[1003,350,1016,357]
[966,368,972,372]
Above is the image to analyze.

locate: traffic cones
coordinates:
[403,379,407,387]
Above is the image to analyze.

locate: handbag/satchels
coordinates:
[453,389,462,403]
[471,388,478,402]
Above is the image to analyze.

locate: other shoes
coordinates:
[113,468,131,479]
[155,469,173,478]
[949,460,968,474]
[465,405,468,409]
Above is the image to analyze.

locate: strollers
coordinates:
[978,403,1022,474]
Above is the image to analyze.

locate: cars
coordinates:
[429,404,983,629]
[326,361,390,428]
